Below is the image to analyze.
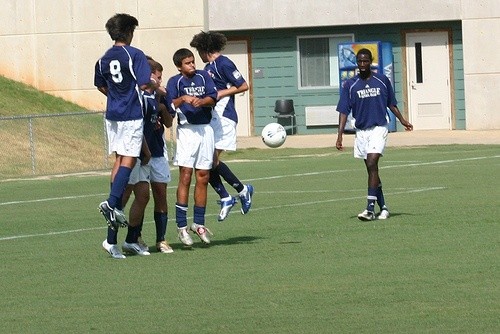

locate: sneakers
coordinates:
[190,223,214,245]
[97,200,119,232]
[156,241,174,254]
[137,236,150,252]
[237,183,254,215]
[216,196,236,222]
[357,209,376,221]
[121,240,151,256]
[113,207,129,228]
[377,209,391,220]
[177,225,194,246]
[102,237,126,259]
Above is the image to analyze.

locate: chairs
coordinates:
[273,99,295,135]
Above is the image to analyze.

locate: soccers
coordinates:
[261,122,287,148]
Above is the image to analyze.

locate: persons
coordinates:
[190,31,253,220]
[102,56,173,258]
[336,48,413,220]
[93,14,156,231]
[164,48,217,246]
